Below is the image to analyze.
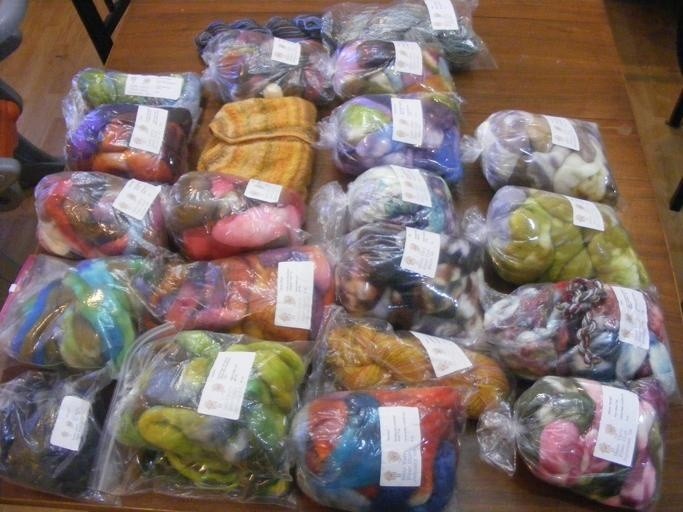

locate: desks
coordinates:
[0,0,683,512]
